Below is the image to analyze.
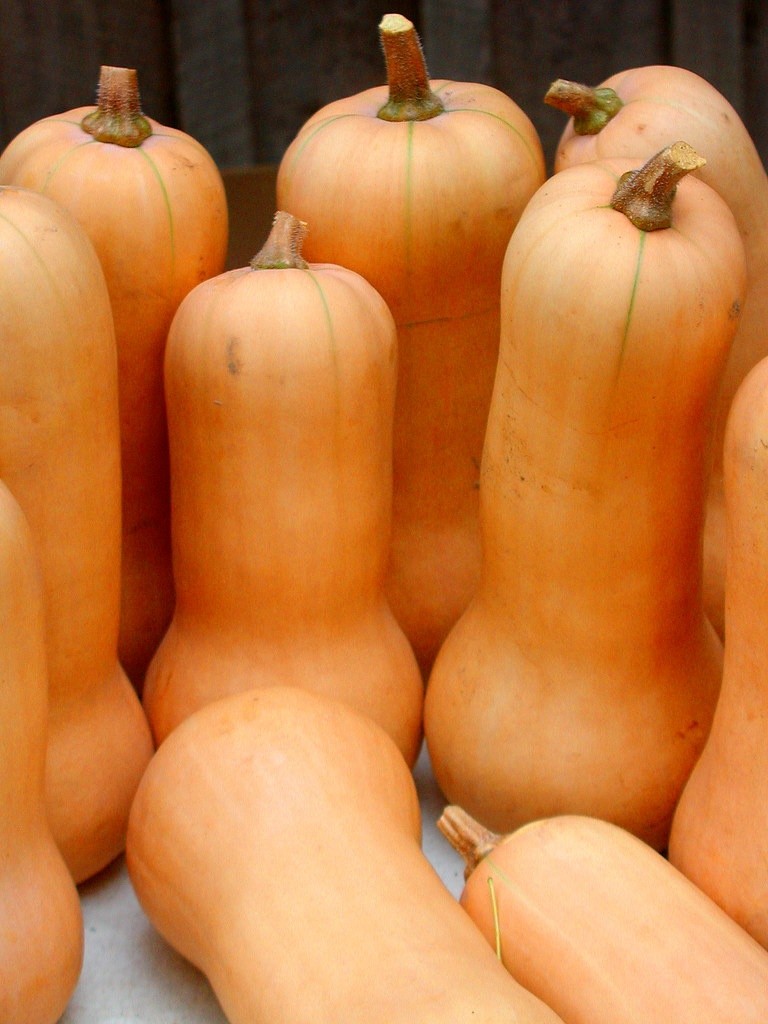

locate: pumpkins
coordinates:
[1,13,768,1024]
